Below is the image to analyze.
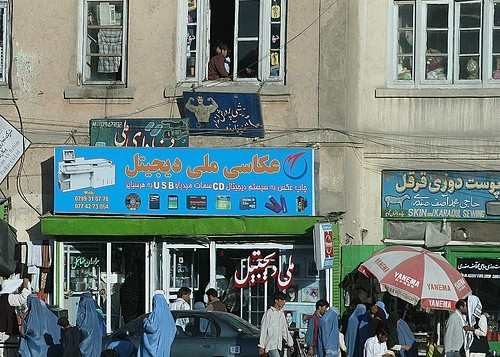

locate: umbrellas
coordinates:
[358,244,472,320]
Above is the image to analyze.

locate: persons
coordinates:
[208,42,231,79]
[137,289,177,357]
[169,286,194,332]
[305,298,490,357]
[257,289,295,356]
[205,287,228,312]
[0,275,123,357]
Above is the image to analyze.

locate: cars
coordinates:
[101,310,261,357]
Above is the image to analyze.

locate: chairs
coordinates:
[185,322,202,334]
